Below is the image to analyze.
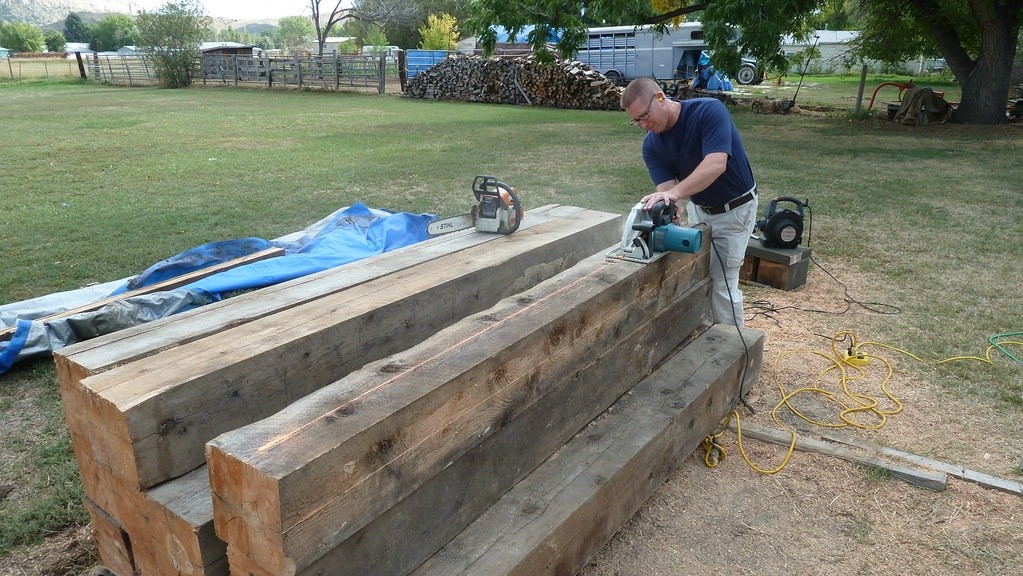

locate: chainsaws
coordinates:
[752,197,809,249]
[605,198,703,264]
[426,176,523,237]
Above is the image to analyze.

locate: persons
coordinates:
[620,77,759,328]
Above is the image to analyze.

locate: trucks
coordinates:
[572,22,767,92]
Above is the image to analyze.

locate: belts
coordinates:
[701,184,758,215]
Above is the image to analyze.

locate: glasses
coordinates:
[630,94,656,126]
